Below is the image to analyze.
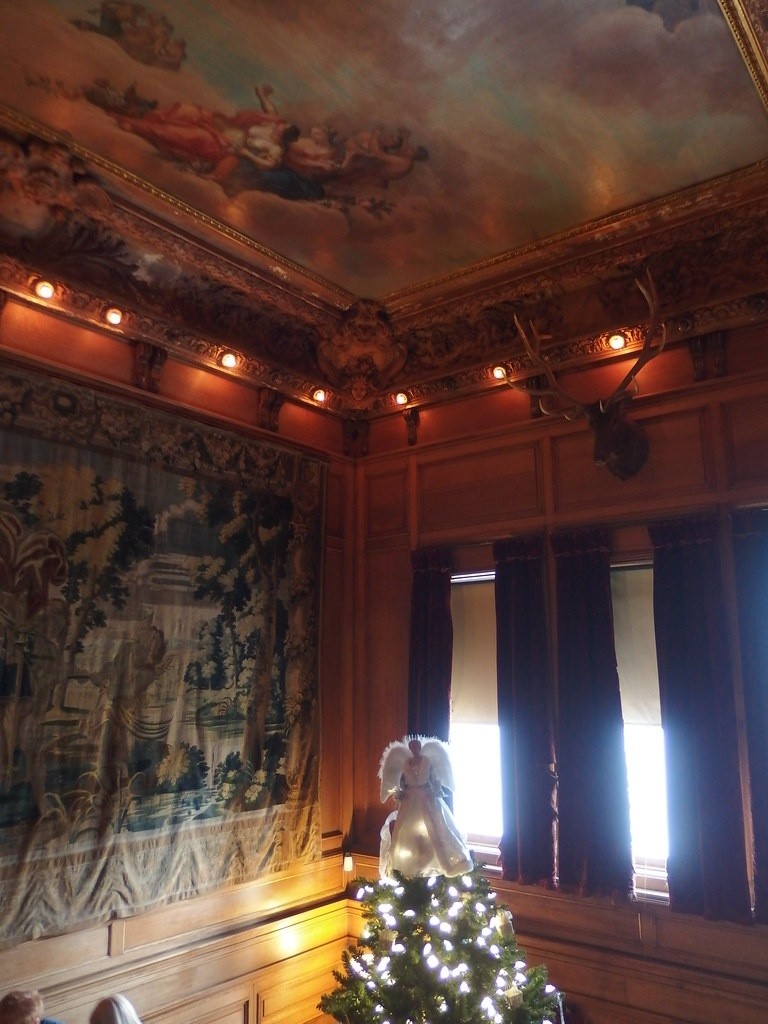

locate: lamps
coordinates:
[344,852,353,871]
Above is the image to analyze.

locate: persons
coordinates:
[0,987,65,1024]
[388,741,472,876]
[88,993,142,1024]
